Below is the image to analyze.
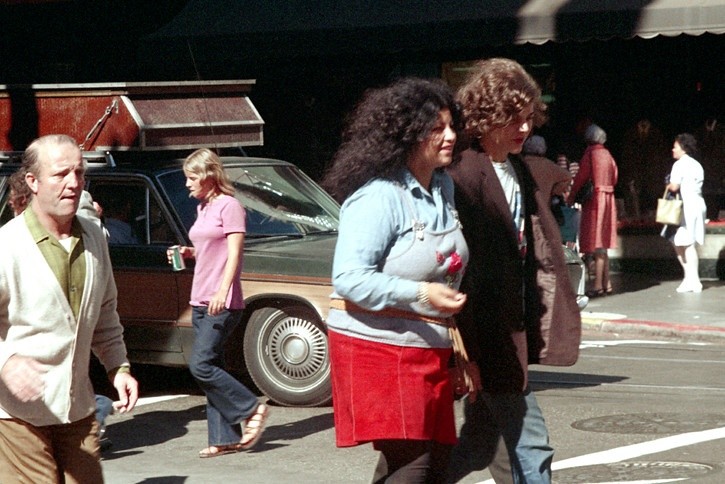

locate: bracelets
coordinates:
[417,280,428,308]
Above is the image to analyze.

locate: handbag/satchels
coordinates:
[655,184,683,227]
[575,149,595,204]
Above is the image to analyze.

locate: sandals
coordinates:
[603,286,614,295]
[585,288,604,297]
[199,443,243,458]
[234,402,270,450]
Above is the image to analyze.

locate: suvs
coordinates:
[5,158,342,406]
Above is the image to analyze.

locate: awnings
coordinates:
[135,2,725,66]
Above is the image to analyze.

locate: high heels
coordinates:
[675,282,703,294]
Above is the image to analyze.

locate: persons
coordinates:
[0,132,138,484]
[656,133,709,294]
[567,119,617,295]
[326,73,470,484]
[72,158,117,461]
[518,130,572,265]
[166,149,270,459]
[9,169,32,217]
[442,58,555,484]
